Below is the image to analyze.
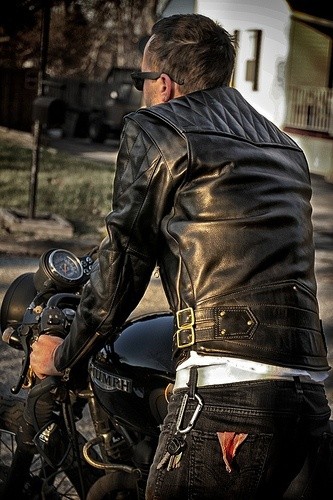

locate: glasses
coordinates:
[131,70,184,91]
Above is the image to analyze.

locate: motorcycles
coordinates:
[0,248,333,500]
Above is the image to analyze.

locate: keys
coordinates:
[155,433,187,472]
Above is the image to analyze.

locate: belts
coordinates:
[172,365,325,394]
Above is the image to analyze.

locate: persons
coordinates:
[30,12,332,500]
